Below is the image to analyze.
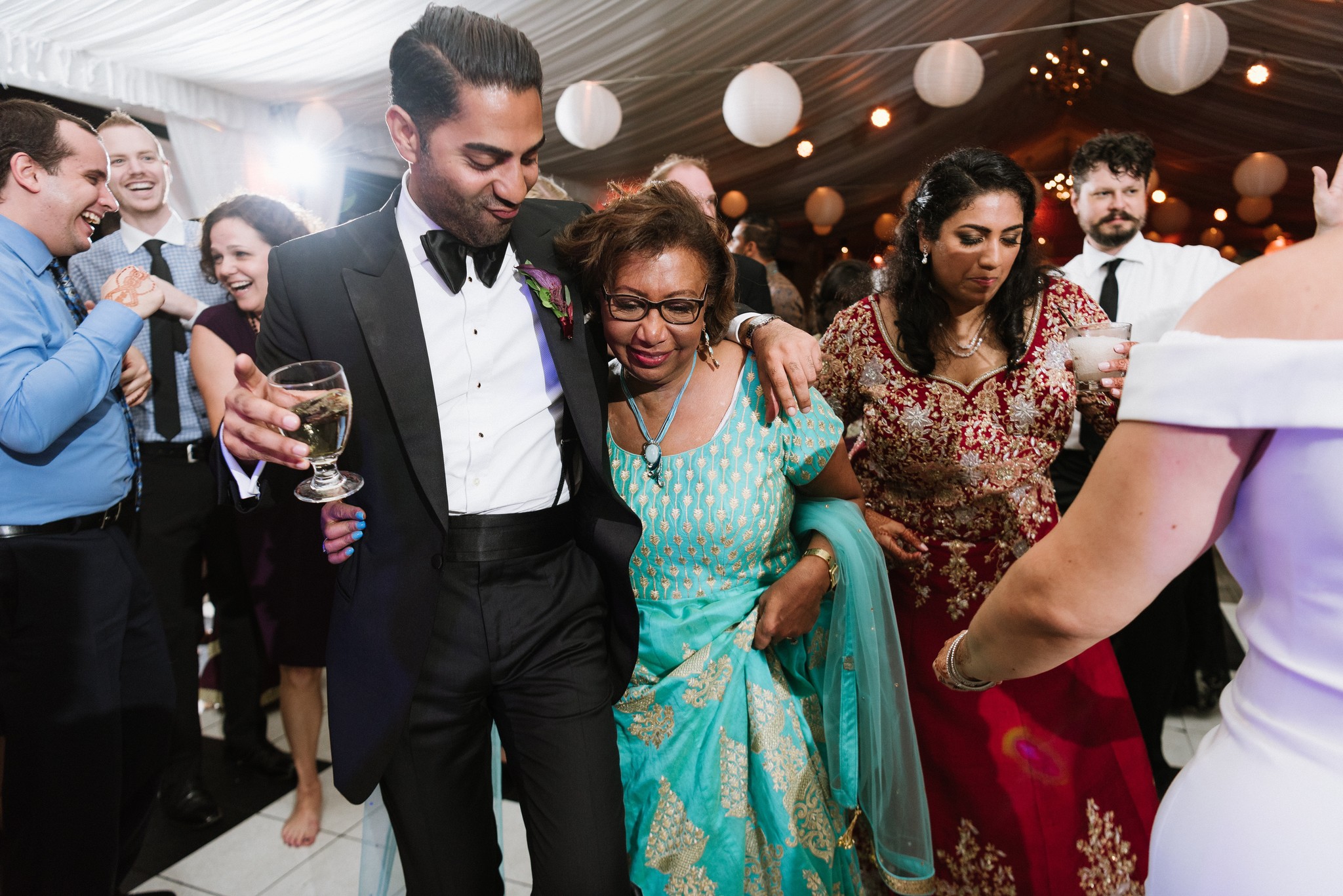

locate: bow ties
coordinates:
[419,229,510,294]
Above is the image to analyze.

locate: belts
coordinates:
[0,502,126,539]
[137,442,207,462]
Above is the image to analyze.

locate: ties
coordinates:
[142,238,189,443]
[1078,258,1126,459]
[46,256,146,513]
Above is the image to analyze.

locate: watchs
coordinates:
[801,547,840,593]
[745,314,784,353]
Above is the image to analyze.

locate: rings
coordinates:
[786,636,799,645]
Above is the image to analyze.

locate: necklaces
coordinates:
[619,349,696,488]
[248,312,262,334]
[936,311,992,358]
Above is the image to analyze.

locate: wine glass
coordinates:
[268,361,366,502]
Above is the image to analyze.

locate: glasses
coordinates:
[601,281,707,325]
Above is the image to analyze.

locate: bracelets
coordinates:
[946,630,1003,692]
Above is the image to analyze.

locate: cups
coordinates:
[1067,323,1133,393]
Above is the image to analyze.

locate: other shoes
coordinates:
[161,782,220,824]
[1168,658,1234,716]
[225,734,290,778]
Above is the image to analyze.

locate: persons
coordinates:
[804,148,1160,896]
[215,3,825,896]
[0,98,179,896]
[320,179,936,896]
[1036,129,1342,800]
[809,258,873,341]
[871,245,899,295]
[67,106,291,834]
[642,153,774,316]
[932,221,1343,896]
[189,194,329,848]
[725,215,807,332]
